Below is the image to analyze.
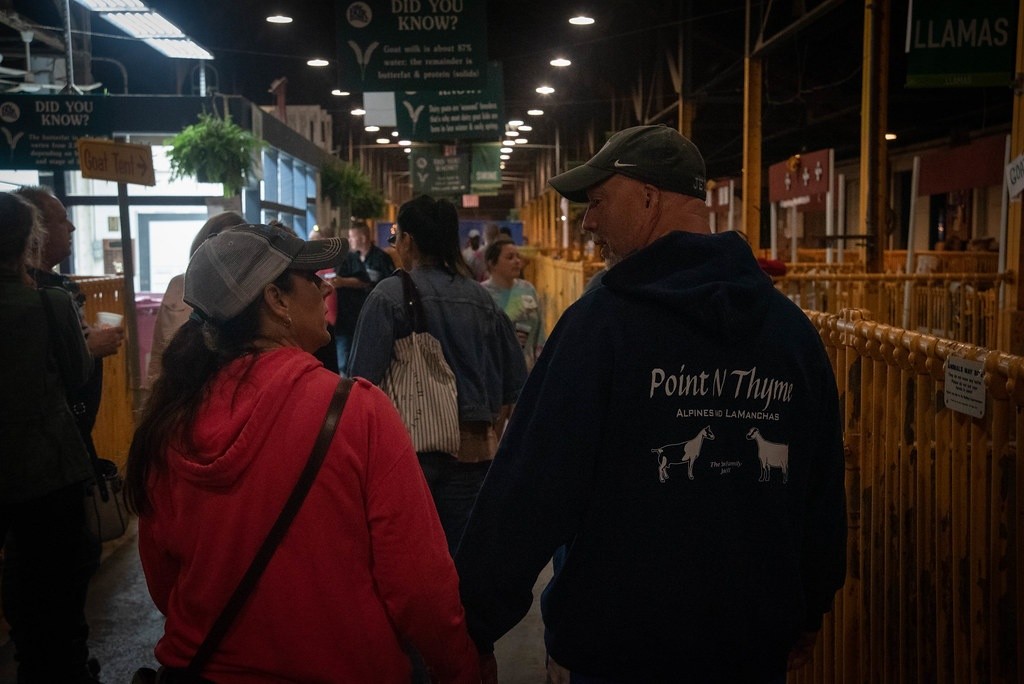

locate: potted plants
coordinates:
[168,110,270,197]
[351,191,383,218]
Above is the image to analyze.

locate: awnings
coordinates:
[918,133,1005,196]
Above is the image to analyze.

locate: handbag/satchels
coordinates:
[80,458,130,543]
[376,268,460,454]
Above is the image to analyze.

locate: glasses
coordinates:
[387,233,414,248]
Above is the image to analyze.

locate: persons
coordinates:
[944,214,997,347]
[455,124,849,684]
[0,188,125,684]
[125,195,546,684]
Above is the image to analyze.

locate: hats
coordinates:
[469,229,481,238]
[547,124,707,203]
[183,224,350,327]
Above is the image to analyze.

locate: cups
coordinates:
[324,272,337,287]
[516,322,532,349]
[96,311,124,328]
